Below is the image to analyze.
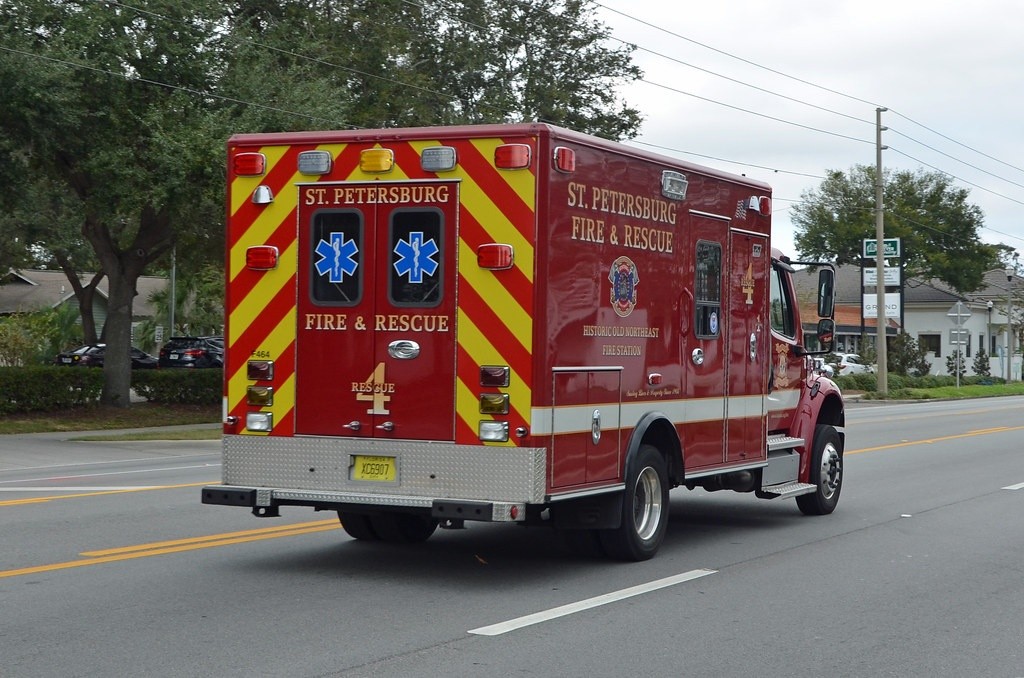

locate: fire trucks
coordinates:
[202,123,845,560]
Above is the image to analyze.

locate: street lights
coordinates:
[1005,266,1015,382]
[987,300,995,358]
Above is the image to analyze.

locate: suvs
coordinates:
[159,337,223,368]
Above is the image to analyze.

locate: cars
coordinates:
[816,351,874,376]
[56,342,158,368]
